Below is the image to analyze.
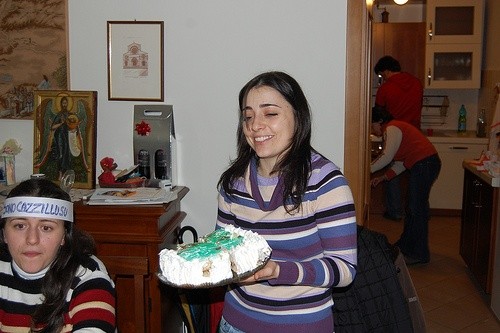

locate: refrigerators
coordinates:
[420,128,489,210]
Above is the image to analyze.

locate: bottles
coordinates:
[458,104,466,132]
[476,107,487,137]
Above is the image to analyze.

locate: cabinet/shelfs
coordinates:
[424,0,486,90]
[372,22,424,82]
[460,169,498,293]
[428,143,488,209]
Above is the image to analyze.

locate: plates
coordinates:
[60,169,75,193]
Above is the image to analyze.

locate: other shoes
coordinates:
[383,210,402,222]
[405,253,430,265]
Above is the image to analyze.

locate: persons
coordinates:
[370,104,441,269]
[1,177,119,333]
[216,70,357,333]
[373,53,424,222]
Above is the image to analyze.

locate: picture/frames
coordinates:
[106,20,164,102]
[32,90,97,189]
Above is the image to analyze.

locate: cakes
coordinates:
[158,224,272,287]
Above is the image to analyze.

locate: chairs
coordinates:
[96,255,149,333]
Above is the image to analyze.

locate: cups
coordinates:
[31,173,46,179]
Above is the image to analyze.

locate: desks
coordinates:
[74,186,191,333]
